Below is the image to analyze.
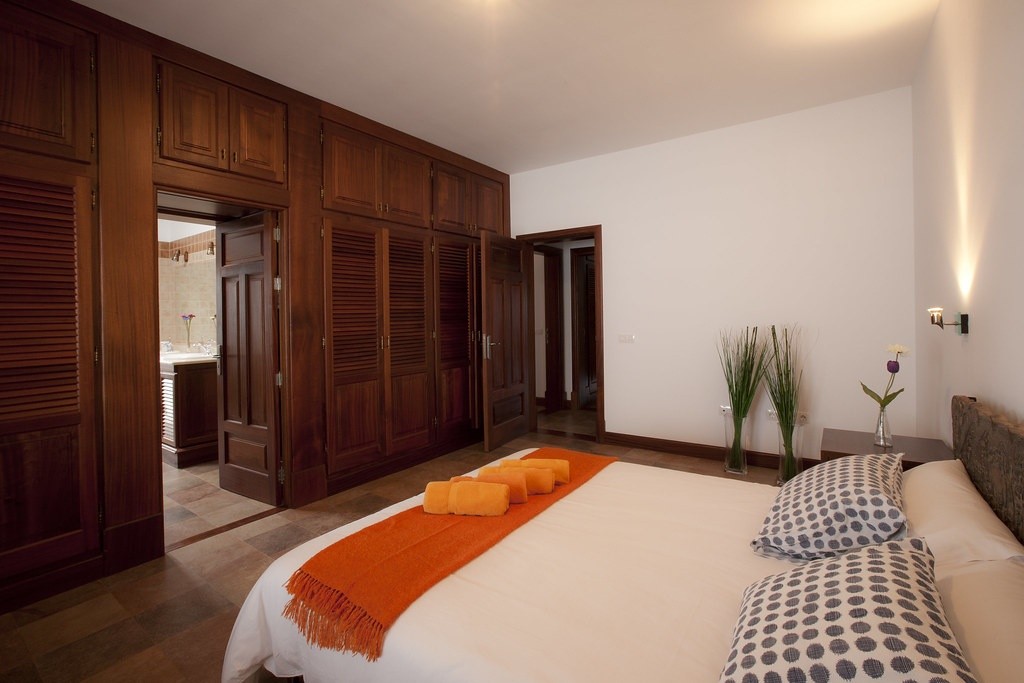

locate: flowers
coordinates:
[859,343,909,407]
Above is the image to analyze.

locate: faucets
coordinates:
[160,336,173,352]
[193,338,216,356]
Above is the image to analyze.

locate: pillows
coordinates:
[719,452,1024,683]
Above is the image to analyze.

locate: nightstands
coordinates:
[820,428,956,472]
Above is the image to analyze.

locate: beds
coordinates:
[218,395,1024,683]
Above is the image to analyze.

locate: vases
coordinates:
[873,410,894,447]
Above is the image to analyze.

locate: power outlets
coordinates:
[720,406,732,416]
[769,410,809,424]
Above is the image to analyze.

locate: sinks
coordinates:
[160,351,216,364]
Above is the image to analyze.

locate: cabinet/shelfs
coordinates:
[0,0,510,616]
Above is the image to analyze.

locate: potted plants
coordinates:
[715,326,774,476]
[758,324,820,487]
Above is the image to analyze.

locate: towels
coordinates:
[479,467,555,494]
[423,481,510,516]
[499,459,570,483]
[450,476,528,503]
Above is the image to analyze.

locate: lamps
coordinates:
[928,308,969,333]
